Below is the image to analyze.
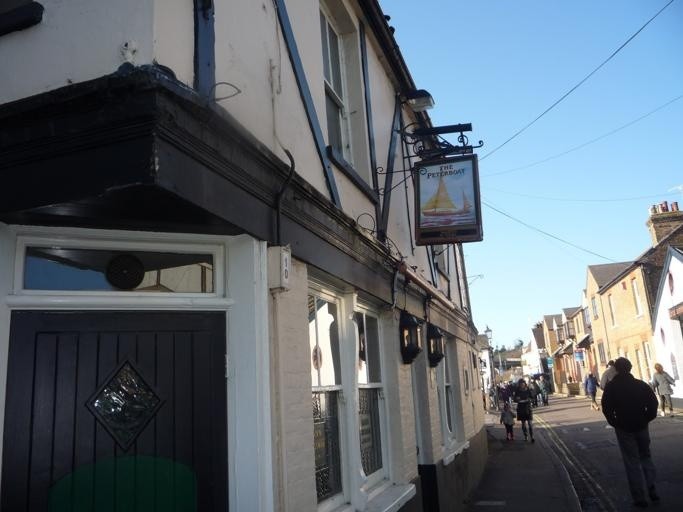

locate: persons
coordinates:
[583,371,602,411]
[648,363,675,417]
[487,370,550,410]
[600,359,618,393]
[498,398,516,440]
[602,357,661,512]
[511,377,537,444]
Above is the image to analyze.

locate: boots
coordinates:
[590,401,601,412]
[506,432,536,443]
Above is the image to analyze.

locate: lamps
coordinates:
[401,310,422,364]
[426,322,444,367]
[397,89,436,112]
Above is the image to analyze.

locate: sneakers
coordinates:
[660,410,675,417]
[633,493,663,507]
